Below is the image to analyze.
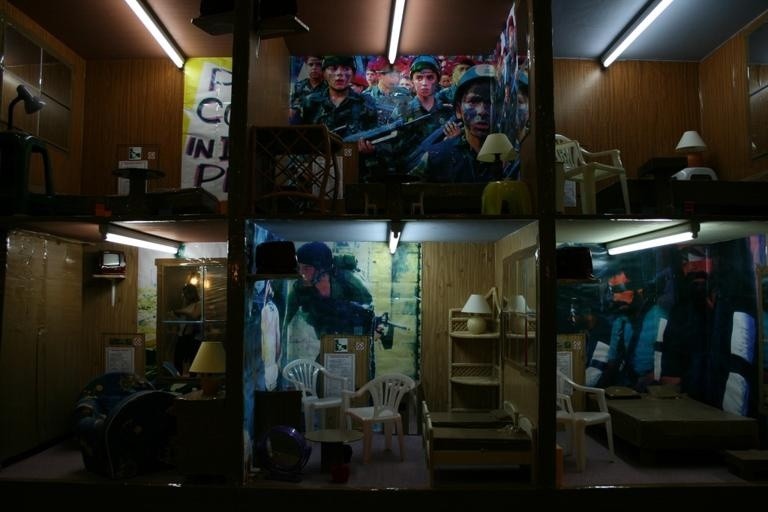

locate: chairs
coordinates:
[409,191,426,216]
[364,192,377,217]
[74,371,158,470]
[555,133,633,216]
[556,369,615,474]
[98,387,178,481]
[254,358,417,474]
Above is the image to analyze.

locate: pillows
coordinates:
[604,386,641,399]
[647,384,680,398]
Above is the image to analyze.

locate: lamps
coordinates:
[460,293,492,335]
[5,84,47,135]
[124,0,186,71]
[675,130,708,168]
[189,340,226,396]
[386,218,409,237]
[383,1,406,65]
[475,132,516,180]
[598,0,659,69]
[503,293,535,335]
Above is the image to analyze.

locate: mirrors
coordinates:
[156,260,204,376]
[500,244,540,377]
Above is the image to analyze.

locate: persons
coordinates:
[175,284,201,320]
[290,15,528,187]
[572,265,713,400]
[283,241,373,484]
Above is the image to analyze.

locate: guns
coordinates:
[343,109,431,146]
[408,115,456,160]
[316,296,407,335]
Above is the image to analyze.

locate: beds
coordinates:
[420,399,540,488]
[585,389,760,467]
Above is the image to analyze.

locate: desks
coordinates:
[174,388,238,485]
[0,134,221,218]
[154,376,204,391]
[657,180,768,222]
[251,122,346,216]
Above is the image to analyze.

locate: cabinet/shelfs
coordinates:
[320,335,373,398]
[2,227,86,465]
[448,286,504,412]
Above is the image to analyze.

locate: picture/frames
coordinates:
[102,332,145,380]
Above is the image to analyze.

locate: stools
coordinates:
[481,179,533,217]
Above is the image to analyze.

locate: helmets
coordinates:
[517,69,529,89]
[168,282,200,309]
[321,54,358,73]
[452,63,497,107]
[296,241,332,271]
[409,55,442,84]
